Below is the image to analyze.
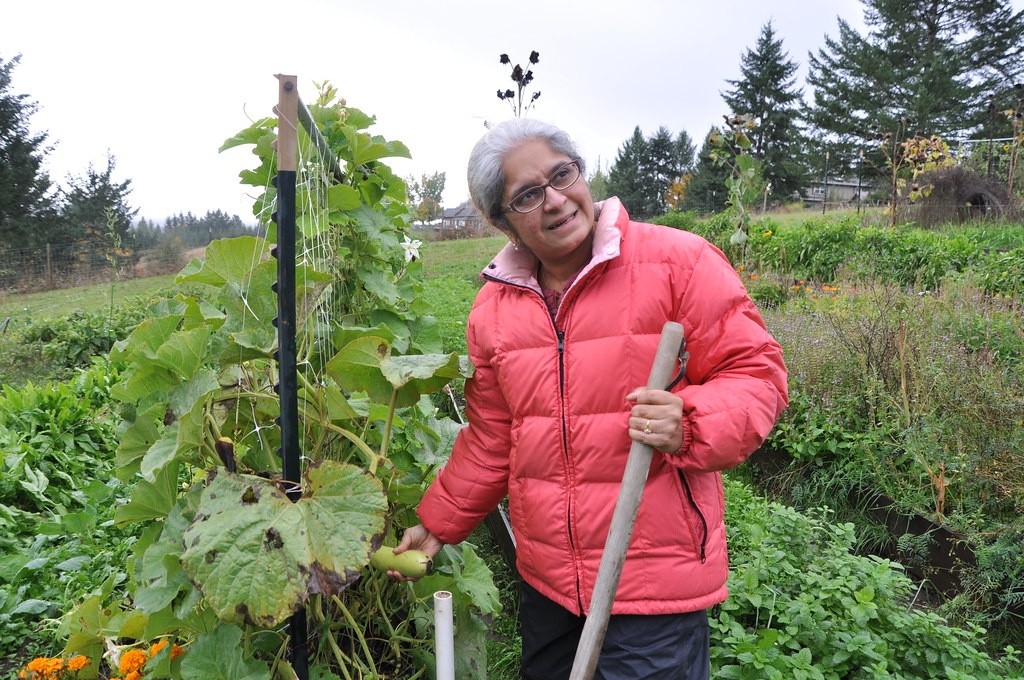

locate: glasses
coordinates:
[501,157,582,216]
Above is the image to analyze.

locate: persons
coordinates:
[387,117,791,680]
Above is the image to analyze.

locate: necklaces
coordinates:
[540,265,552,286]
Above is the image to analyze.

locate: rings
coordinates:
[644,420,652,432]
